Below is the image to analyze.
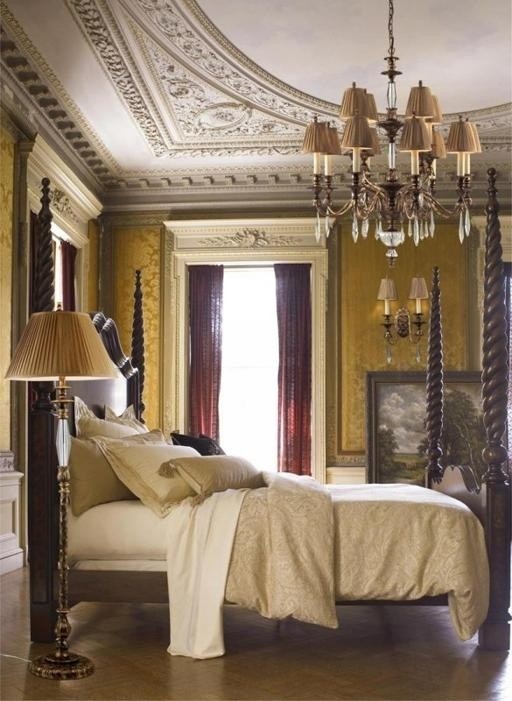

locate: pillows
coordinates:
[68,395,265,521]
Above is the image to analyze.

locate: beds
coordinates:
[30,167,512,659]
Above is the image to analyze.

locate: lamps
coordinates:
[301,0,482,269]
[3,303,119,679]
[376,273,430,345]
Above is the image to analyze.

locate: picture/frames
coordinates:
[367,369,488,550]
